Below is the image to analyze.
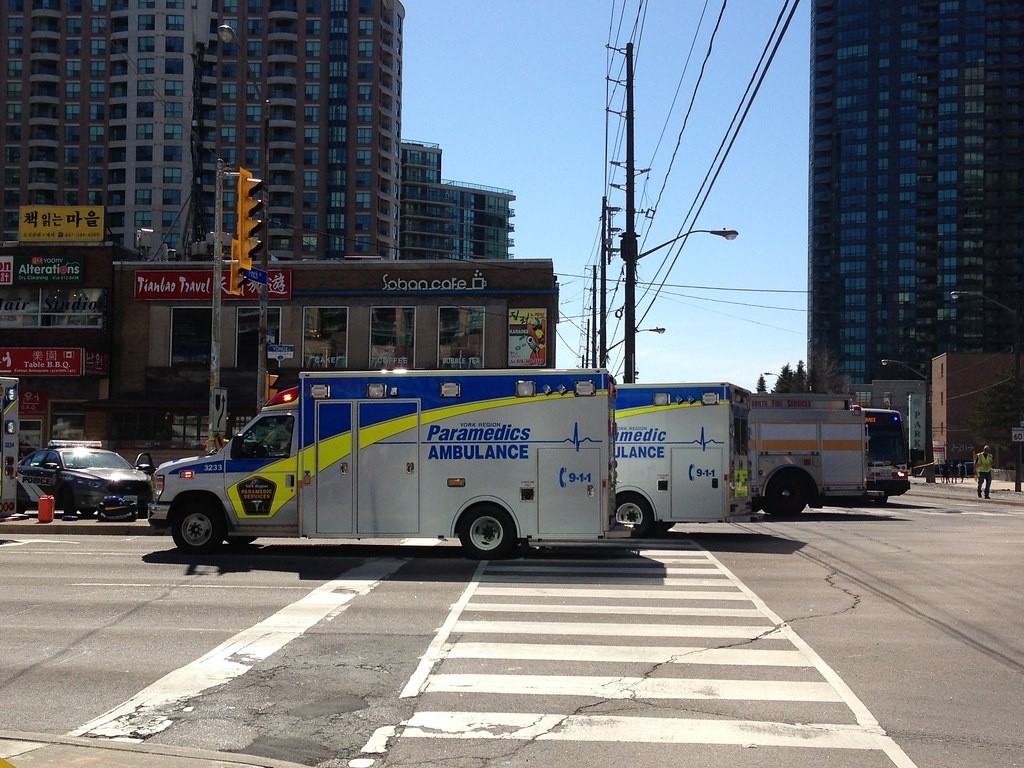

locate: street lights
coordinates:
[625,228,740,384]
[598,328,667,369]
[951,289,1023,493]
[217,24,272,416]
[880,359,933,470]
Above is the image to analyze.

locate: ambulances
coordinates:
[616,382,757,540]
[746,385,886,519]
[148,367,637,557]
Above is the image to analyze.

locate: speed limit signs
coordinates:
[1011,426,1024,443]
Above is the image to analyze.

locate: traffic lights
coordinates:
[230,238,246,297]
[239,168,262,269]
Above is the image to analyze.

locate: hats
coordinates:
[983,445,990,451]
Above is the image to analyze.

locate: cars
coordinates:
[15,438,159,519]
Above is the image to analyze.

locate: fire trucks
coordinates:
[0,377,19,524]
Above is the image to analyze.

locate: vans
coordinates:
[861,403,913,501]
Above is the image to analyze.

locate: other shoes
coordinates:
[978,492,982,497]
[985,496,990,499]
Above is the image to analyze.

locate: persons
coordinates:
[940,459,965,483]
[975,446,993,499]
[268,421,293,456]
